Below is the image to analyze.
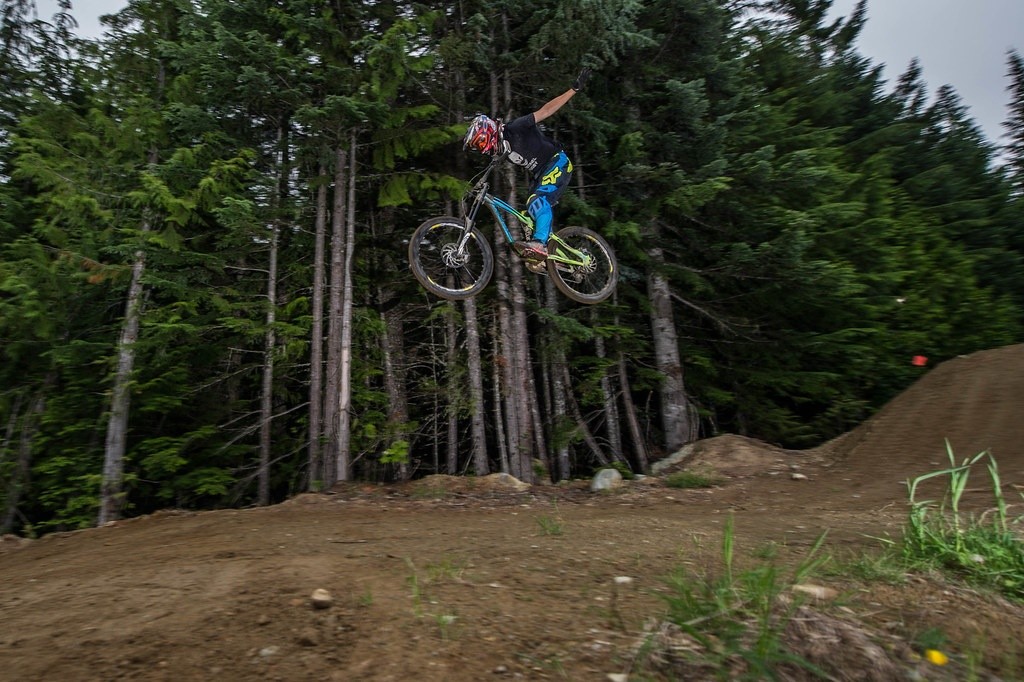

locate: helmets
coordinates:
[462,114,500,159]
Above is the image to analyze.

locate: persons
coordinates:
[463,64,592,261]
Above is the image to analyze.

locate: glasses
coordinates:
[471,133,487,150]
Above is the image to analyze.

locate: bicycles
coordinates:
[407,150,619,303]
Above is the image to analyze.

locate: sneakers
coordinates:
[525,261,547,274]
[513,240,548,261]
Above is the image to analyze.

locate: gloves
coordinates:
[573,65,595,93]
